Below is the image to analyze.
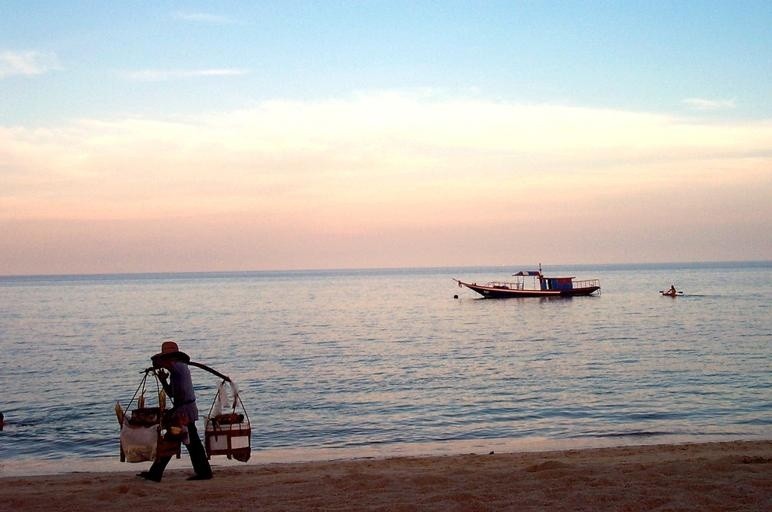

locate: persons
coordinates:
[136,342,215,482]
[668,285,676,295]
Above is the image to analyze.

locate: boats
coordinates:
[663,291,683,297]
[453,264,601,298]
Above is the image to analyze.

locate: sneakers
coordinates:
[141,470,162,482]
[186,474,213,480]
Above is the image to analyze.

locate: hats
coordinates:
[151,342,190,363]
